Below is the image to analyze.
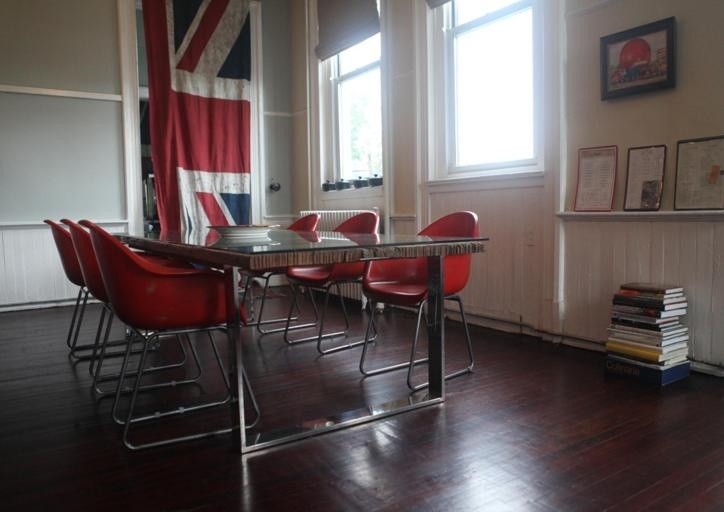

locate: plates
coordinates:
[205,224,282,239]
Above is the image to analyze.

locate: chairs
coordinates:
[283,211,380,356]
[58,219,202,397]
[242,213,322,335]
[44,220,156,362]
[77,221,261,449]
[358,211,478,392]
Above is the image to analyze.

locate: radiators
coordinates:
[300,206,385,314]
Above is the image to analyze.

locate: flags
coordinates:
[142,0,253,268]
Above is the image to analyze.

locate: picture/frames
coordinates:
[601,15,674,103]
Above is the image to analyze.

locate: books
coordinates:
[606,282,691,388]
